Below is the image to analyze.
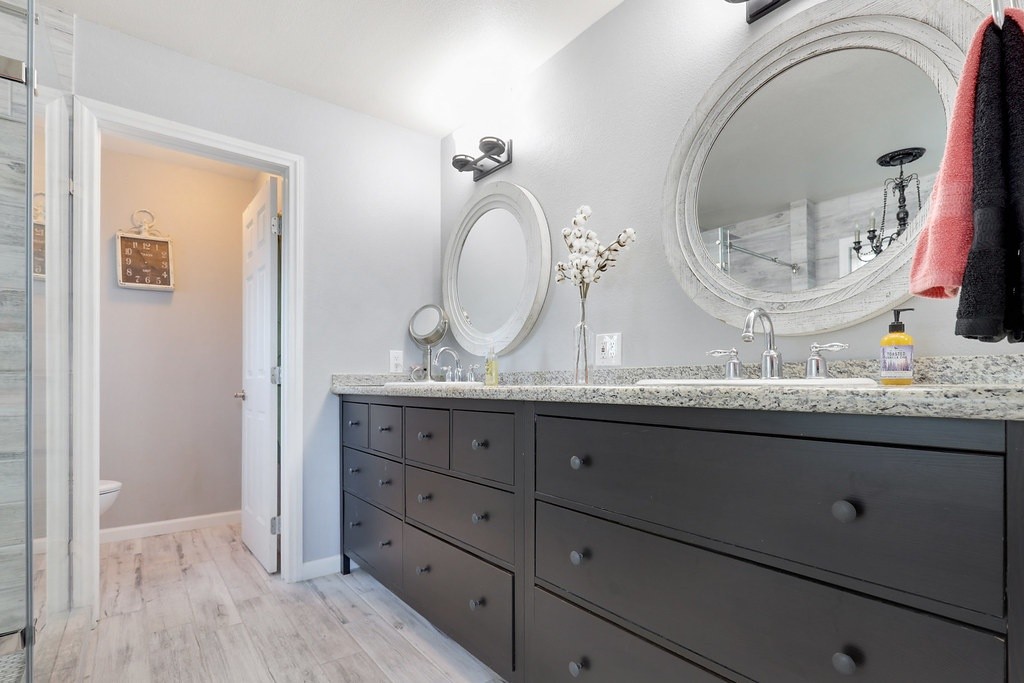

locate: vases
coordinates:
[573,323,593,386]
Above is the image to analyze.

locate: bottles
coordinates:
[486,346,498,387]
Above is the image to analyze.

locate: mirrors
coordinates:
[660,0,987,338]
[442,181,553,360]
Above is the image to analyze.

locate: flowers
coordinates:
[555,206,636,301]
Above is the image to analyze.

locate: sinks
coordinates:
[385,382,484,387]
[635,377,878,387]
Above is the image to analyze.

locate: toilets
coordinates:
[99,480,122,517]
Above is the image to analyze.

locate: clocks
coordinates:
[116,231,176,292]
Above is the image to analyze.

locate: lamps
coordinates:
[852,147,927,263]
[451,136,512,182]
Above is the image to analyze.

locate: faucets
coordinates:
[433,347,463,382]
[741,307,784,379]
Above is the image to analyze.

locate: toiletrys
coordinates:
[483,345,499,386]
[879,307,916,385]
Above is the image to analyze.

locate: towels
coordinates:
[954,15,1024,344]
[907,5,1024,300]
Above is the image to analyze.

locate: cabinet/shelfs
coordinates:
[338,393,1024,683]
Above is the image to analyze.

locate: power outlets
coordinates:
[595,332,621,366]
[389,350,404,374]
